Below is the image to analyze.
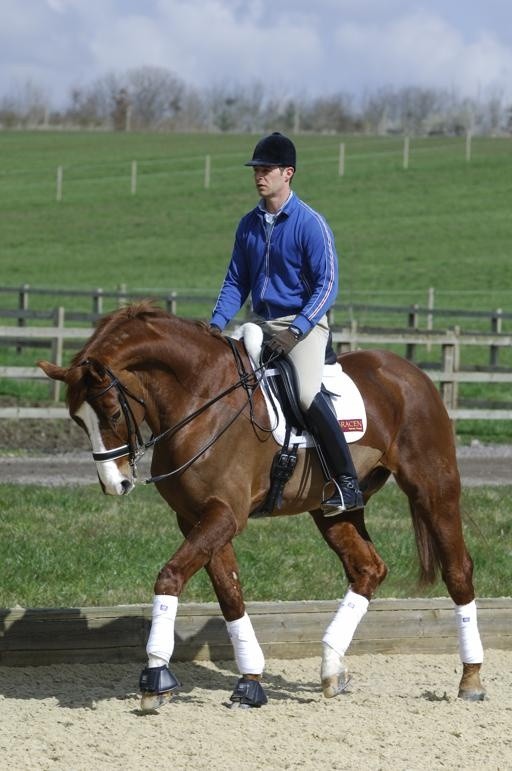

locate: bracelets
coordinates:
[287,324,302,339]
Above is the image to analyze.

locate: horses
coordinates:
[37,304,490,704]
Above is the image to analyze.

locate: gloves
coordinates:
[263,327,302,364]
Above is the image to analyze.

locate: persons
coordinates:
[210,131,369,518]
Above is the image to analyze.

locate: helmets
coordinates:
[245,132,296,168]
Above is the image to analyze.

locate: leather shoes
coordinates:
[321,473,365,513]
